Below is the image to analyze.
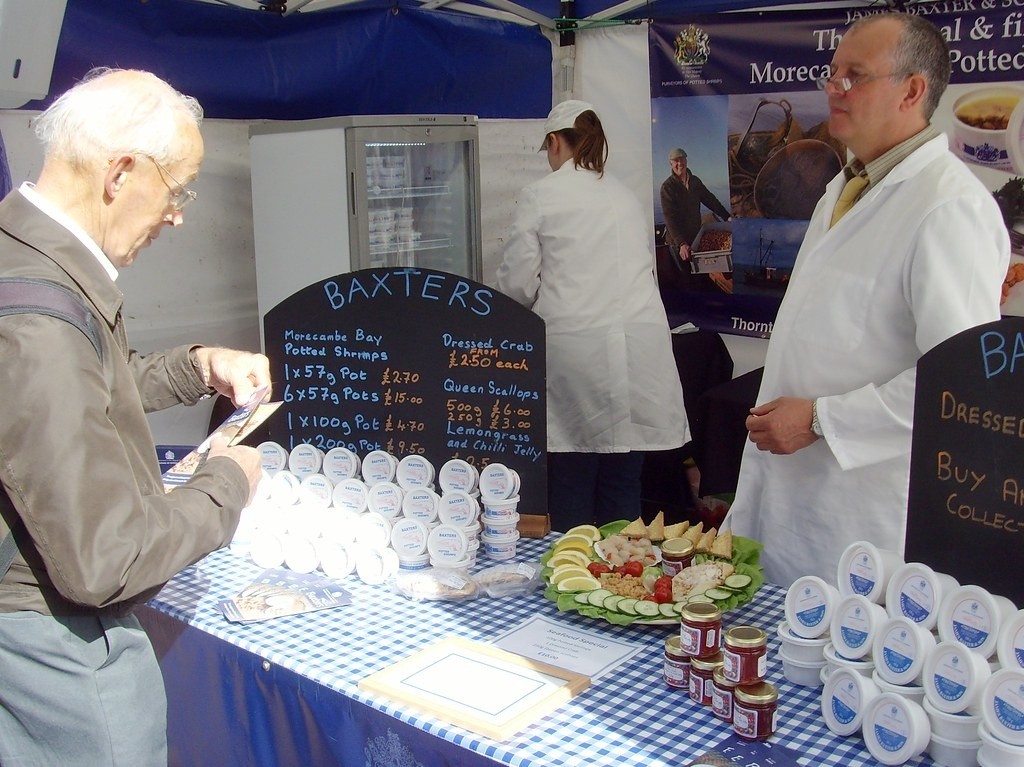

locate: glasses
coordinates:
[111,151,197,214]
[816,71,898,92]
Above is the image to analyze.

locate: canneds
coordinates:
[663,603,778,741]
[659,537,696,579]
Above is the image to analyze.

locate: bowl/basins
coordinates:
[952,87,1024,171]
[777,541,1024,767]
[479,464,520,560]
[235,441,406,585]
[427,459,480,572]
[391,455,441,573]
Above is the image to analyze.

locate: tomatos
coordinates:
[653,576,673,603]
[587,561,642,578]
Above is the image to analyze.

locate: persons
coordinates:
[714,12,1011,591]
[496,100,693,533]
[660,147,733,273]
[0,60,272,767]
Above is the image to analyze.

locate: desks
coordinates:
[129,475,1024,767]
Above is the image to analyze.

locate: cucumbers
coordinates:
[575,575,752,618]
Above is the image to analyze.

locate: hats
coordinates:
[537,100,597,154]
[669,148,687,160]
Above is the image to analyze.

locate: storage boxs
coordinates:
[688,219,734,278]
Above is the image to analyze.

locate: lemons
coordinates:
[548,525,603,592]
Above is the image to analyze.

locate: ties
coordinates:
[828,177,869,231]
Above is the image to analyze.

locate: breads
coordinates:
[396,567,530,600]
[621,510,733,559]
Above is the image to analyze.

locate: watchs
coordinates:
[810,397,825,439]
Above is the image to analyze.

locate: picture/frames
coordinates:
[363,636,591,742]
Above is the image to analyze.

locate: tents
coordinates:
[1,0,1024,119]
